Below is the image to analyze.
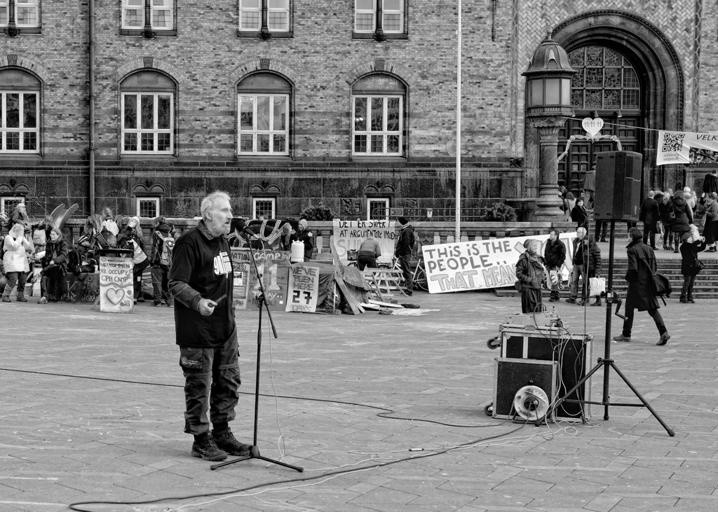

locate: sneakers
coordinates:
[37,296,46,304]
[2,295,11,302]
[17,295,26,302]
[613,335,630,342]
[192,436,227,461]
[655,332,669,345]
[211,428,251,456]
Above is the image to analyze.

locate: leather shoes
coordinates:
[679,299,687,303]
[548,291,600,306]
[687,298,693,303]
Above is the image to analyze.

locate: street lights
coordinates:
[518,21,580,227]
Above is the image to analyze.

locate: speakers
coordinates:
[594,150,643,222]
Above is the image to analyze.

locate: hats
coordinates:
[523,239,531,248]
[398,217,408,225]
[680,233,690,241]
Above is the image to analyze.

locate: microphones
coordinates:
[235,221,258,240]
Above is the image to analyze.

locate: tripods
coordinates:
[535,221,674,437]
[210,240,304,472]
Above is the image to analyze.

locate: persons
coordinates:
[396,216,415,296]
[168,191,250,462]
[515,240,545,313]
[614,227,670,345]
[292,219,312,263]
[679,233,706,302]
[0,201,181,307]
[543,231,566,302]
[279,223,295,252]
[642,185,718,255]
[559,185,608,306]
[357,239,382,270]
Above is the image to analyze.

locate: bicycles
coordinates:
[379,251,431,293]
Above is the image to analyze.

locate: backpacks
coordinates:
[154,229,175,268]
[399,228,421,257]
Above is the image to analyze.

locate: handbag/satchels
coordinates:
[694,260,704,272]
[647,274,670,296]
[588,276,605,297]
[667,211,674,221]
